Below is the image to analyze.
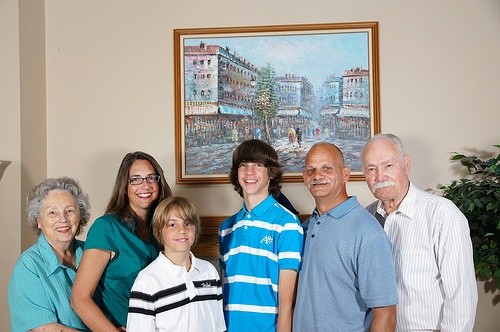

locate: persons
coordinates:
[291,142,399,332]
[362,133,478,332]
[69,151,172,332]
[126,196,226,332]
[8,178,90,332]
[219,140,304,332]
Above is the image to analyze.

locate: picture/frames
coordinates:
[173,22,382,184]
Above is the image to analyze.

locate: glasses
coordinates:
[128,175,160,185]
